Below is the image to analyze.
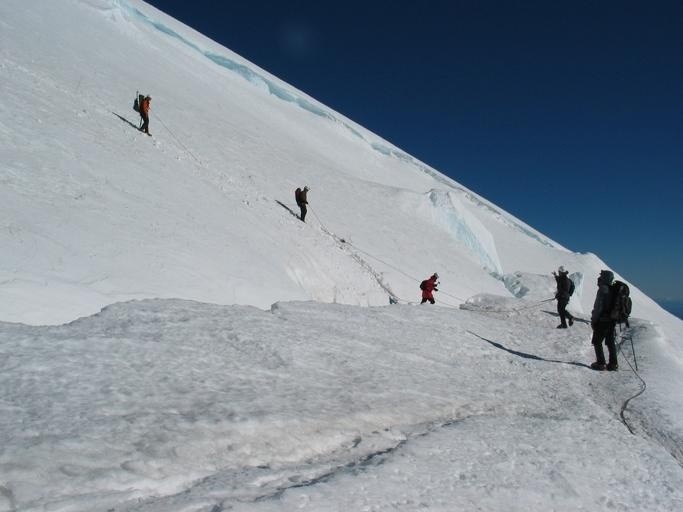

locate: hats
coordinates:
[148,94,153,99]
[304,184,311,191]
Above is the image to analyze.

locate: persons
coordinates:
[553,266,574,328]
[299,185,309,222]
[591,270,618,370]
[420,273,439,304]
[138,95,153,136]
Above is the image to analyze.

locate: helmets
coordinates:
[558,266,566,272]
[434,272,439,278]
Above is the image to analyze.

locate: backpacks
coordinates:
[570,278,575,295]
[295,188,301,205]
[420,280,426,291]
[609,280,632,322]
[133,95,143,112]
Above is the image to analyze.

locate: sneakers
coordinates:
[556,317,575,329]
[591,360,617,371]
